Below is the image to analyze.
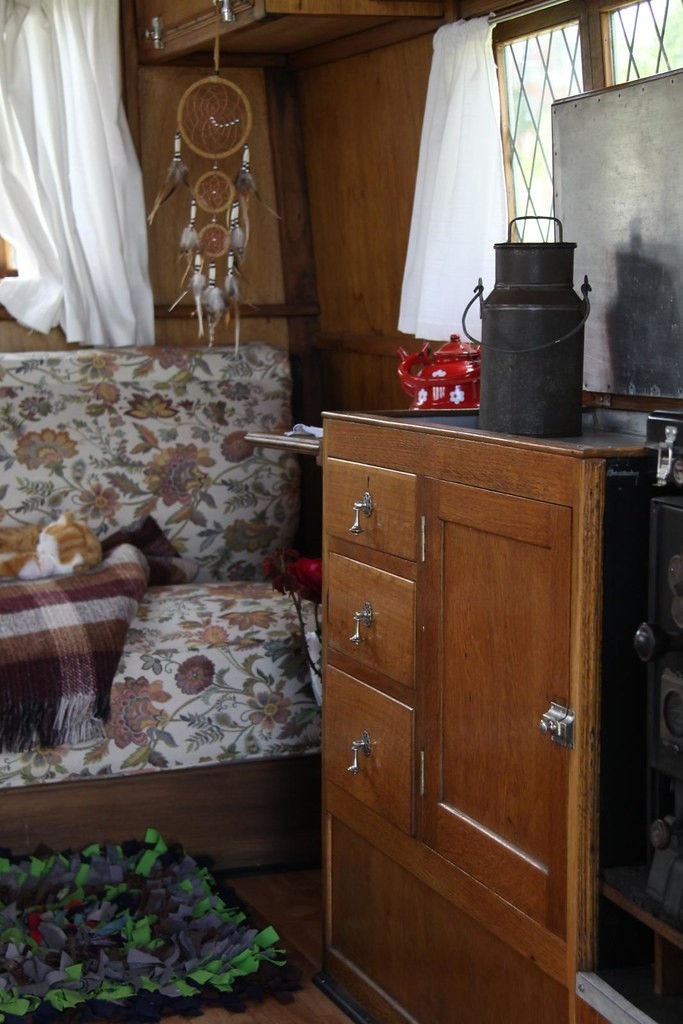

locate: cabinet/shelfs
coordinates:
[319,399,683,1024]
[135,0,457,68]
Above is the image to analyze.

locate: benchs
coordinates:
[1,341,327,873]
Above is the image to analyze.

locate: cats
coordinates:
[0,512,102,580]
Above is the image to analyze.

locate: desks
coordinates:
[243,431,324,464]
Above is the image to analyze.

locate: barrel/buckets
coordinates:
[464,215,590,436]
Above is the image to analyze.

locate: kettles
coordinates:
[396,334,480,409]
